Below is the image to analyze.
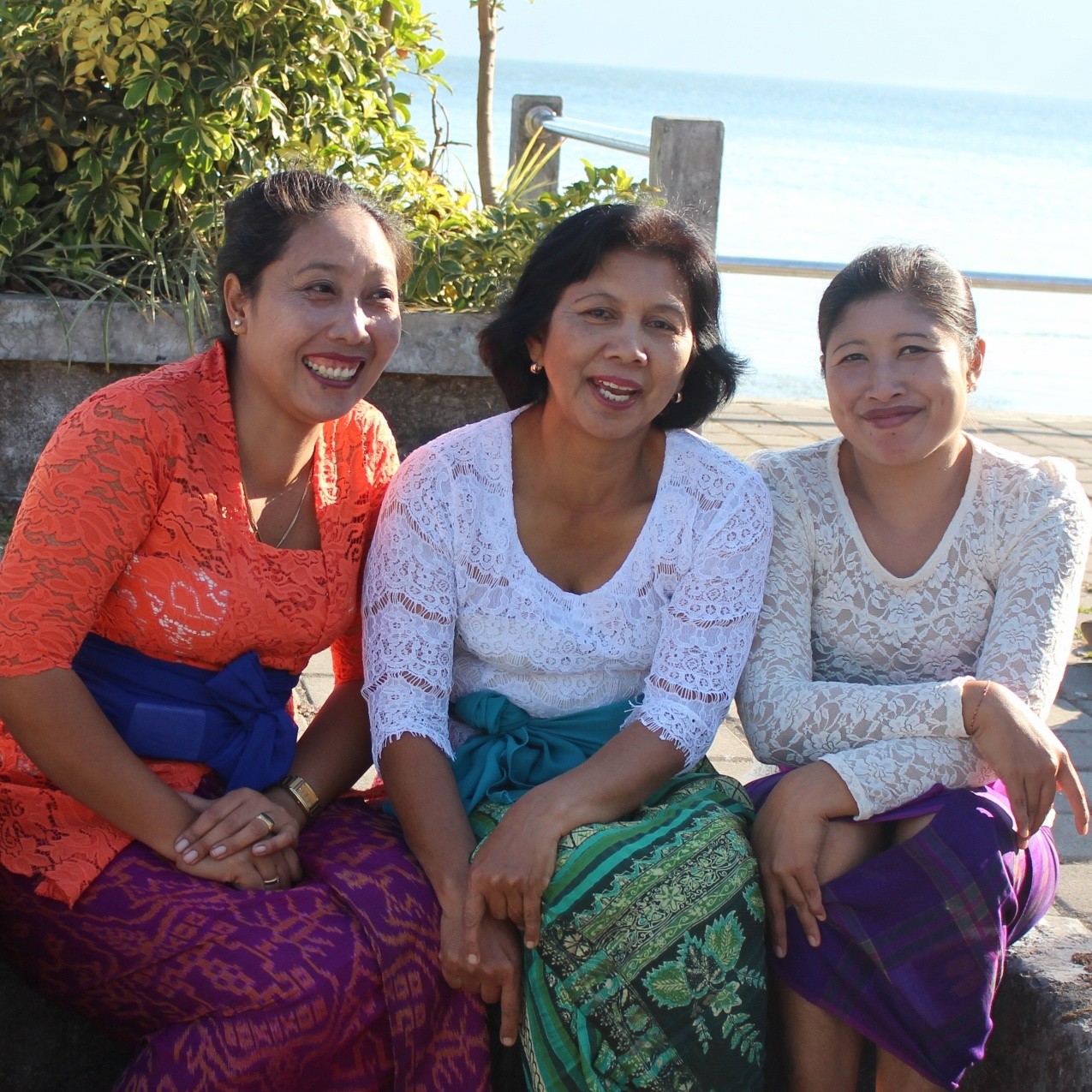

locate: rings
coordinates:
[264,877,279,885]
[257,813,275,830]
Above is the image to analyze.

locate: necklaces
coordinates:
[241,467,314,548]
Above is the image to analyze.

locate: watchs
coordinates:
[280,775,320,827]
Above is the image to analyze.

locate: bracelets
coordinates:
[970,680,991,734]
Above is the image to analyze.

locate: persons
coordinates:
[359,202,772,1092]
[728,243,1092,1092]
[0,169,493,1092]
